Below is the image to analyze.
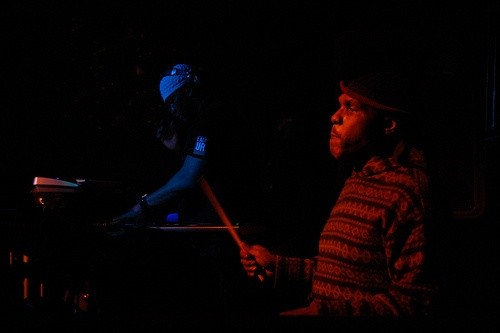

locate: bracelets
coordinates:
[139,190,149,210]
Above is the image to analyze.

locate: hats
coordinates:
[339,54,446,119]
[160,63,197,101]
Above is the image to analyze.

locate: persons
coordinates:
[114,64,233,226]
[241,77,424,318]
